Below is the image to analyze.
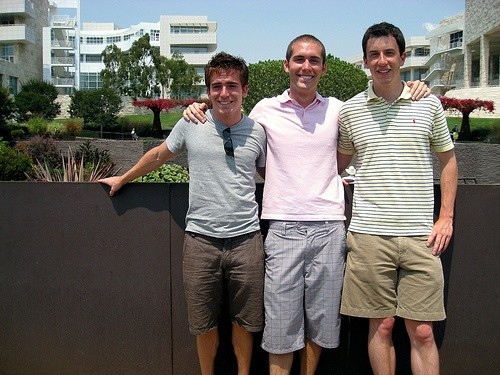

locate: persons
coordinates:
[97,51,349,375]
[337,22,458,375]
[183,34,431,375]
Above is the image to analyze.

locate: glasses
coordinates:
[222,127,234,156]
